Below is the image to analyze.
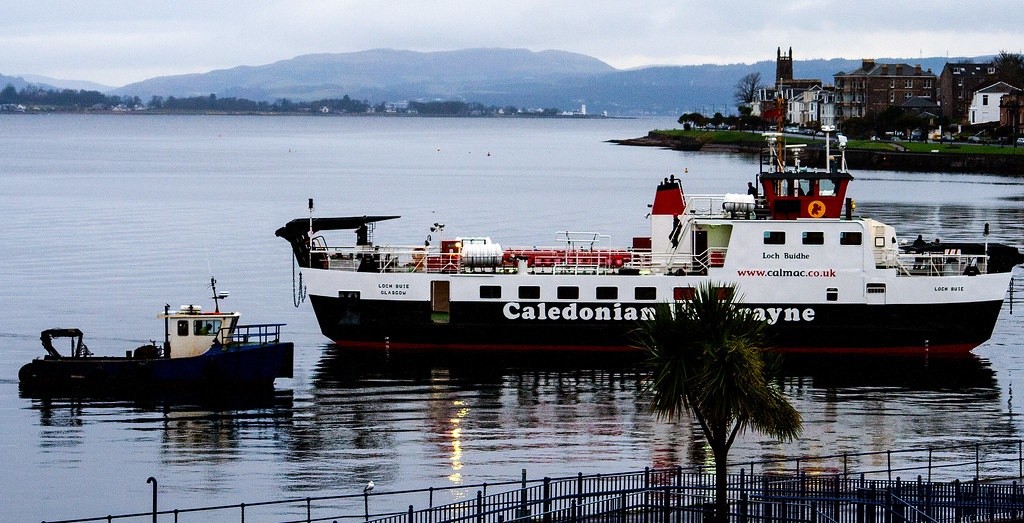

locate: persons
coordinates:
[200,323,213,335]
[748,182,758,216]
[912,235,927,270]
[929,238,945,271]
[668,213,682,250]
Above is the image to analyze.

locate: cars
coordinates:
[968,136,981,144]
[784,124,826,137]
[870,131,957,143]
[1016,138,1024,146]
[695,122,738,130]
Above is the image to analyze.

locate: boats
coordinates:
[274,72,1024,376]
[18,273,294,403]
